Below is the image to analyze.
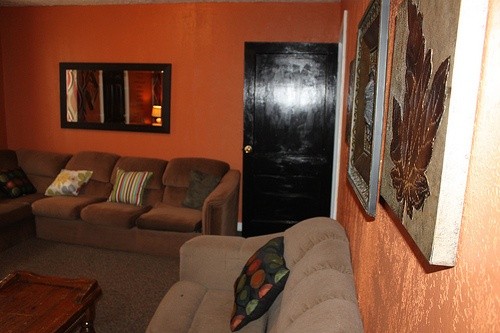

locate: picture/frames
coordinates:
[345,0,390,217]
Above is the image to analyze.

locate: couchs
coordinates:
[0,148,240,254]
[145,217,362,333]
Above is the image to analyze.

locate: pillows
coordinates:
[106,167,153,208]
[231,236,289,332]
[45,169,93,196]
[0,167,36,198]
[183,170,221,210]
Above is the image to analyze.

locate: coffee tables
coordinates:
[0,271,103,333]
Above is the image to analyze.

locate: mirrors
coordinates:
[59,61,172,134]
